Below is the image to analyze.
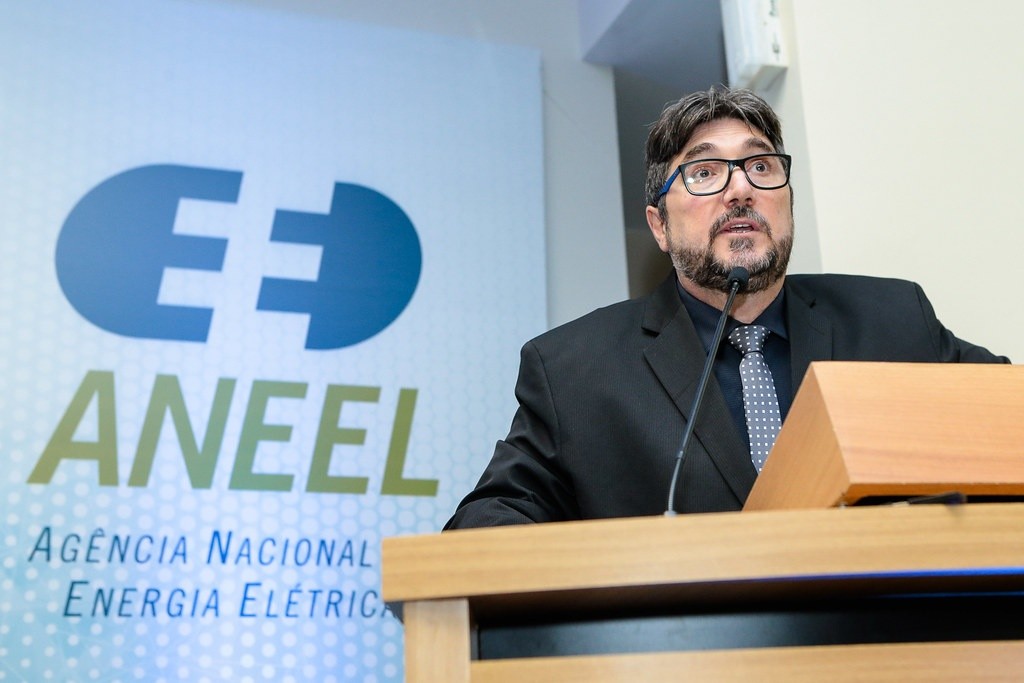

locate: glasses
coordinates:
[659,153,791,196]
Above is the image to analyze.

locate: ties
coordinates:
[729,325,782,475]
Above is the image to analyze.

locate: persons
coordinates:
[439,88,1017,534]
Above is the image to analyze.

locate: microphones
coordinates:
[664,267,750,516]
